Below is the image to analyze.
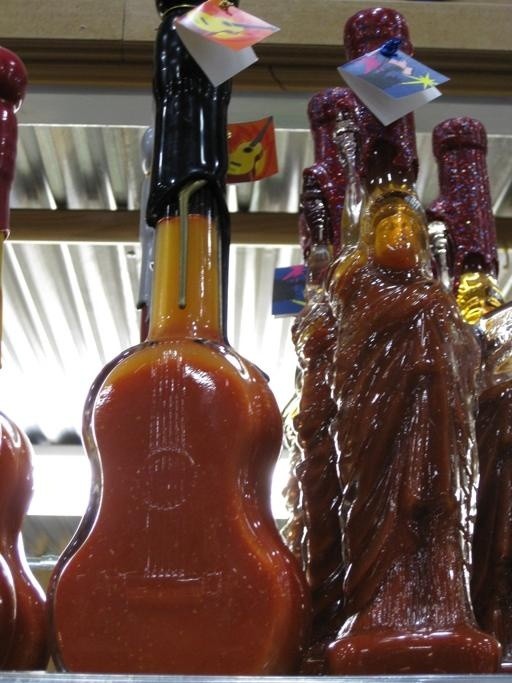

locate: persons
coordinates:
[290,179,483,630]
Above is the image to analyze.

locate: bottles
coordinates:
[51,0,314,673]
[3,40,49,668]
[286,3,510,674]
[136,128,162,347]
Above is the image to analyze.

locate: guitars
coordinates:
[228,116,272,178]
[188,11,276,37]
